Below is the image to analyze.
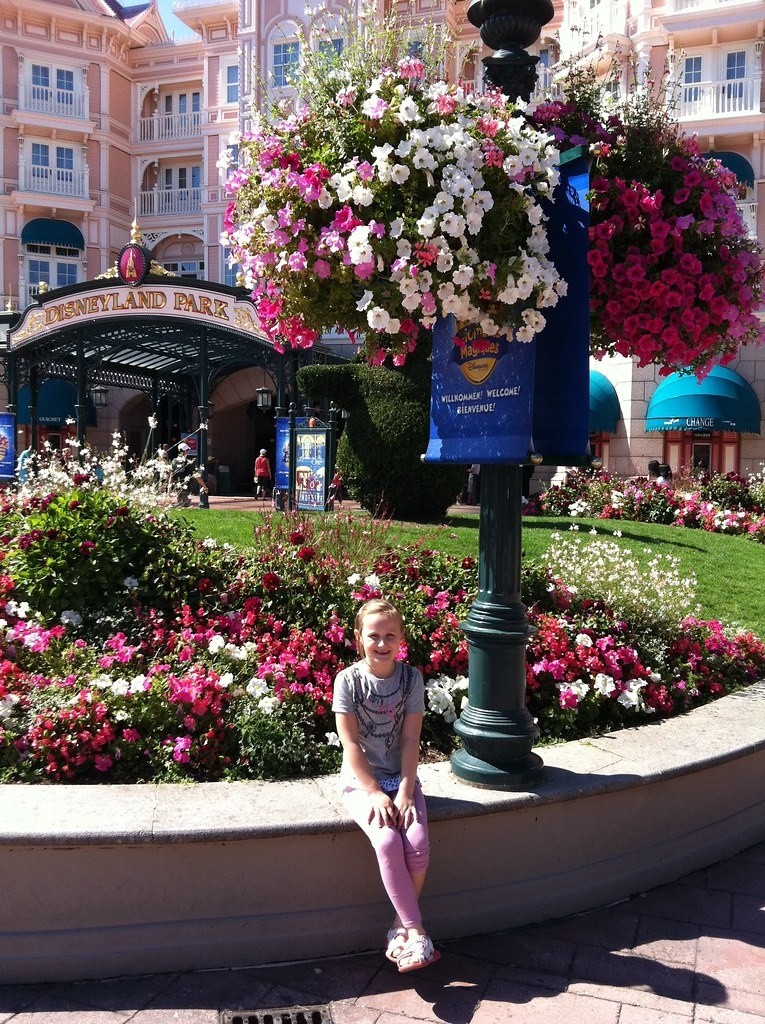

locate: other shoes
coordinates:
[338,506,344,509]
[261,499,267,501]
[254,494,259,500]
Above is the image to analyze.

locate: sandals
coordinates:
[385,921,441,973]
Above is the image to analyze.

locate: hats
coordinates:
[260,449,267,454]
[177,443,191,452]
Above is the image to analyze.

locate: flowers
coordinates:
[533,9,764,386]
[212,0,567,365]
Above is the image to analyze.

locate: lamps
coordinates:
[206,400,215,420]
[90,344,109,412]
[256,355,272,413]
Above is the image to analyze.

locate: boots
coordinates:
[466,496,472,504]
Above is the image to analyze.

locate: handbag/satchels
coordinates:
[253,477,259,483]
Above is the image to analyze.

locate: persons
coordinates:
[648,460,673,490]
[331,599,443,974]
[168,443,208,508]
[253,448,271,502]
[327,465,344,510]
[17,444,32,483]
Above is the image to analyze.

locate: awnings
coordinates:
[14,411,98,426]
[644,364,761,434]
[589,371,619,434]
